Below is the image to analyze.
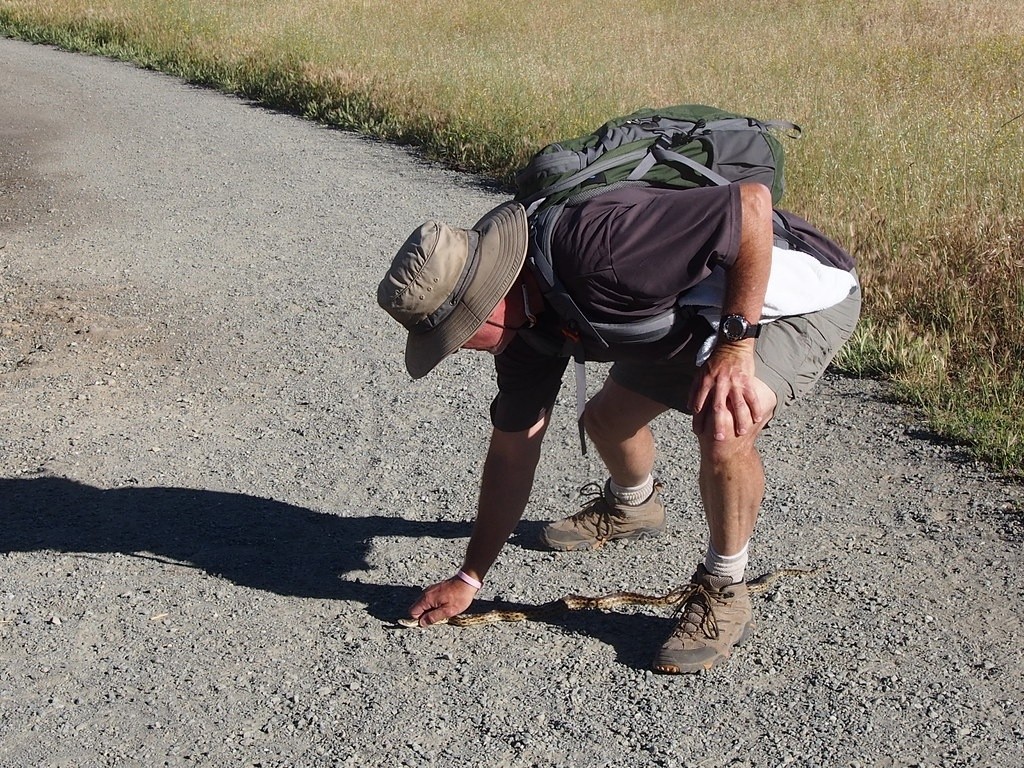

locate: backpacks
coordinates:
[515,103,802,349]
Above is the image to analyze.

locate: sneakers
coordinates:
[539,477,667,552]
[652,563,755,676]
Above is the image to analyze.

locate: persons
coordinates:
[374,179,862,672]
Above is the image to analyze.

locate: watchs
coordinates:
[717,312,762,341]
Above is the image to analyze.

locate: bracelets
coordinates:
[456,570,484,590]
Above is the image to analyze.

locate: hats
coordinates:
[377,200,530,380]
